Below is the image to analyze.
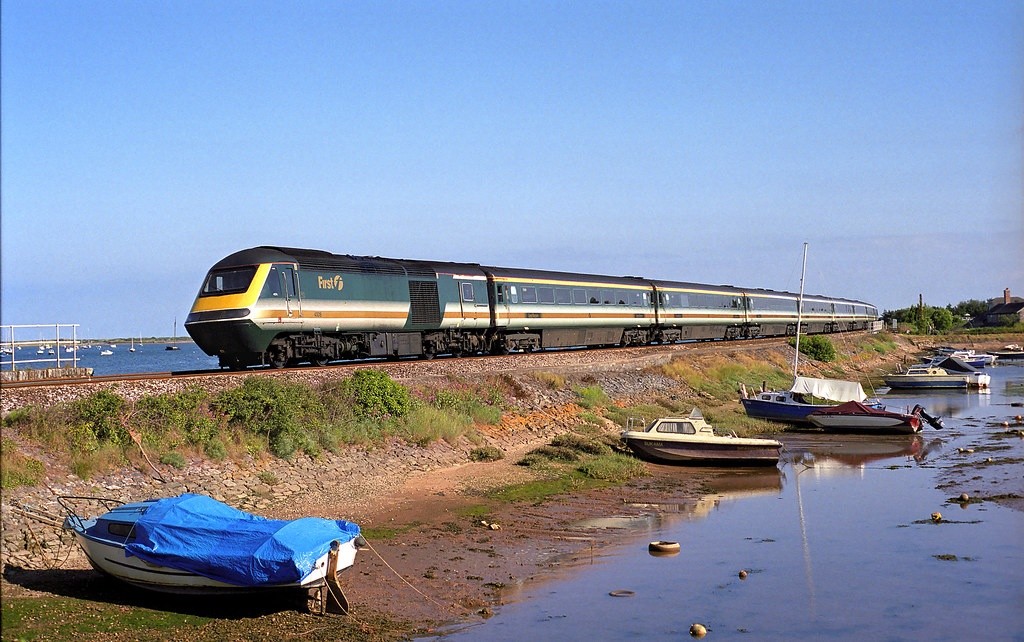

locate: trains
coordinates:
[184,244,878,366]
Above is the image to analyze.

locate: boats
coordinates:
[986,343,1024,358]
[620,408,784,466]
[881,355,991,390]
[57,495,362,592]
[931,345,999,365]
[919,351,986,368]
[100,349,114,356]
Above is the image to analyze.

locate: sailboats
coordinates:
[0,314,180,358]
[738,241,947,433]
